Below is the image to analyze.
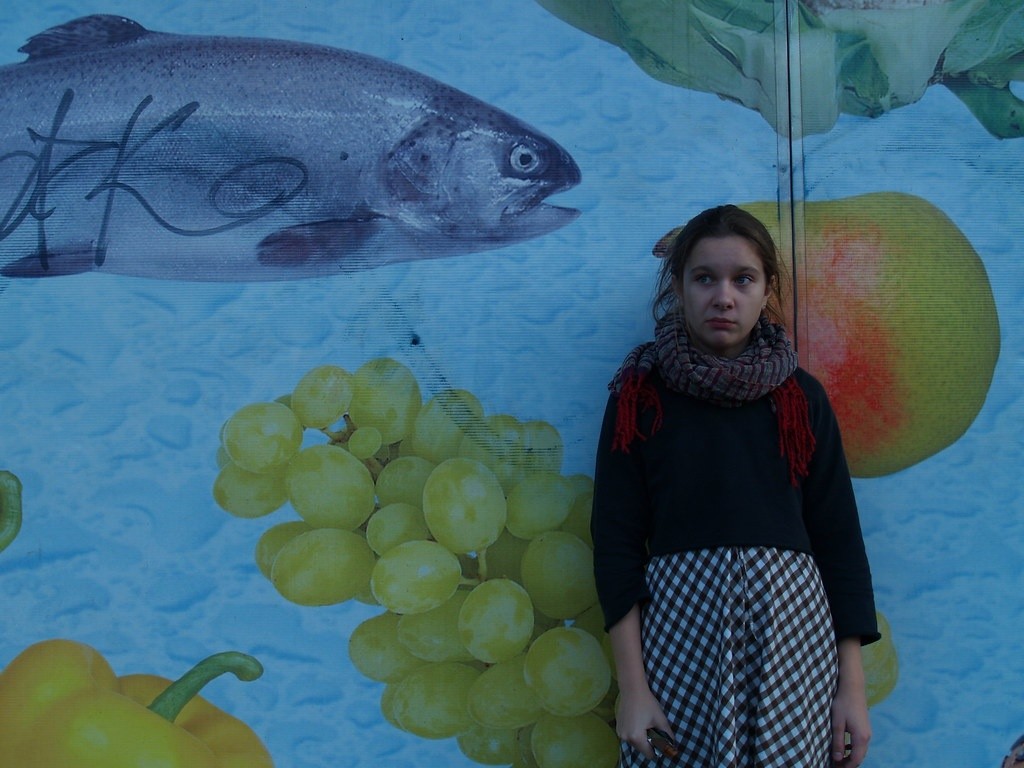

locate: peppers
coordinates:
[0,638,276,768]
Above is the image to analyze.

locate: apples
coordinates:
[742,194,1000,478]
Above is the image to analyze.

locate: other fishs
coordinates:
[0,14,582,284]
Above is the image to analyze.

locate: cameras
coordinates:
[646,729,678,760]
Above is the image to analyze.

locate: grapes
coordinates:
[213,360,898,767]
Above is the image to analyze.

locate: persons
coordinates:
[590,205,883,767]
